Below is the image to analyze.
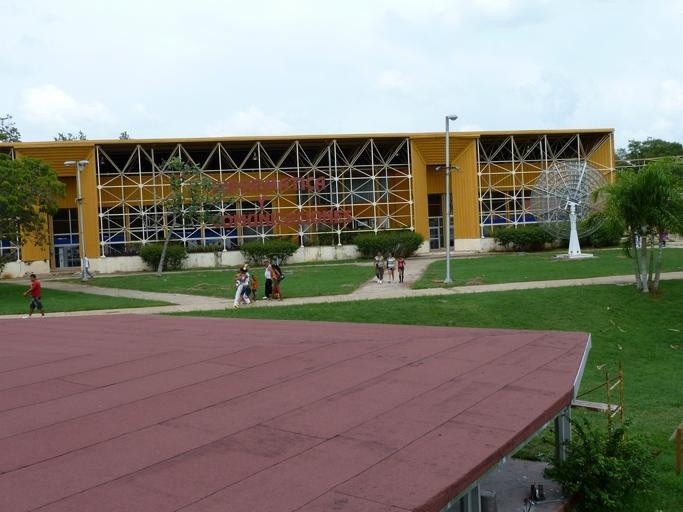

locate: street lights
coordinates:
[436,115,462,285]
[62,158,91,283]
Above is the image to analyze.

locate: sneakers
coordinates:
[377,278,394,283]
[232,294,284,308]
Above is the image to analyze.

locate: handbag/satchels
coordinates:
[277,273,285,282]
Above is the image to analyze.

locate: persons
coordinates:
[396,255,406,283]
[21,273,46,317]
[385,251,396,283]
[231,260,285,308]
[659,232,669,247]
[372,250,385,285]
[635,231,641,249]
[80,253,93,279]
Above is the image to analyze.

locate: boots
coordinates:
[398,275,404,283]
